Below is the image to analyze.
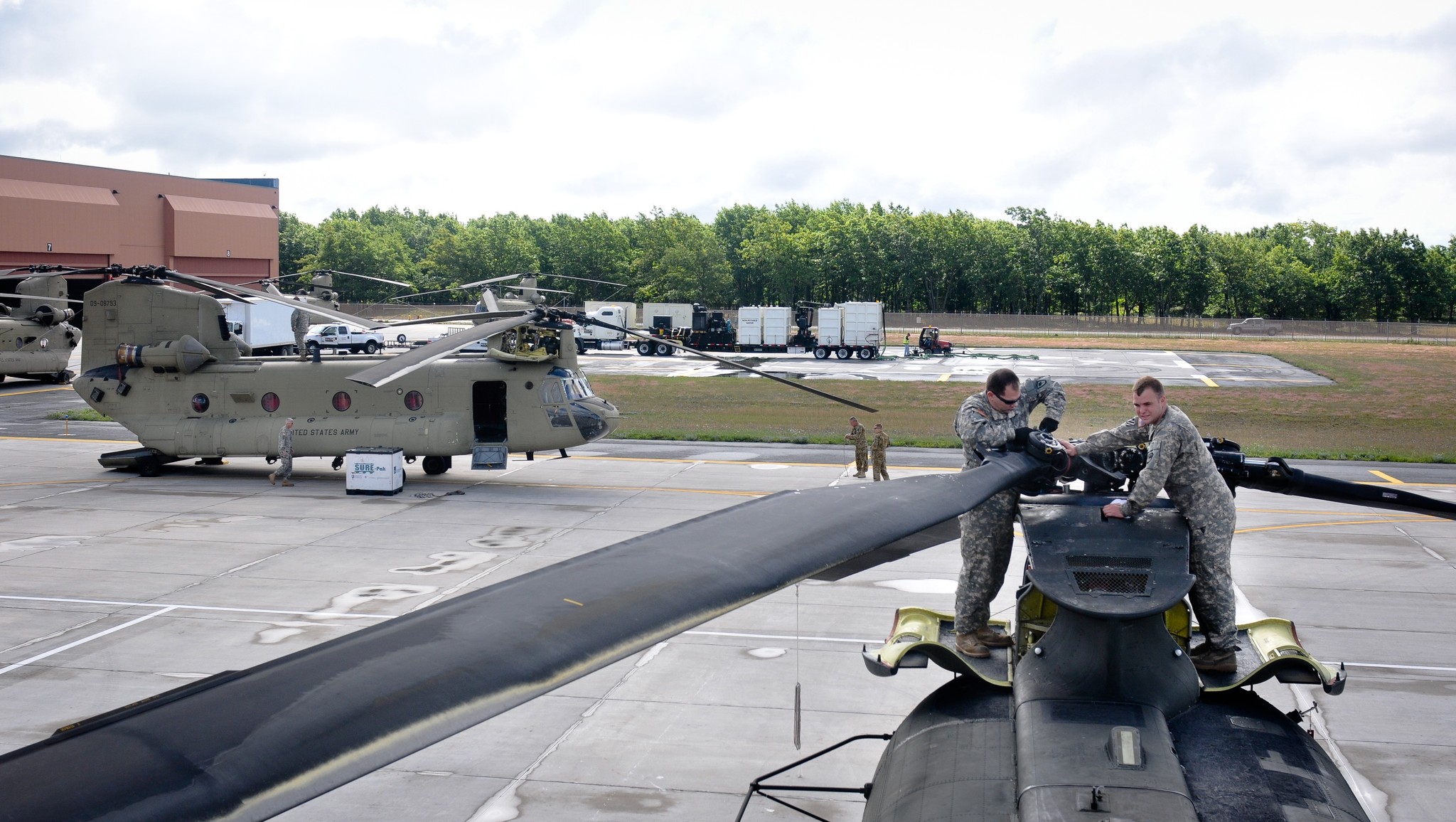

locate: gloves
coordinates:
[1013,427,1036,443]
[1038,416,1059,434]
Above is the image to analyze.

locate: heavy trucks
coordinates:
[556,306,691,357]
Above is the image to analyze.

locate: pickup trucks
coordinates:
[306,322,384,355]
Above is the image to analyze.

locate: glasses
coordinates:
[874,428,881,429]
[991,390,1021,405]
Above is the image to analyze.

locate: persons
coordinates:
[903,333,910,357]
[269,418,294,486]
[291,297,310,361]
[845,416,869,478]
[926,332,934,346]
[1058,377,1242,671]
[953,368,1066,658]
[871,423,890,481]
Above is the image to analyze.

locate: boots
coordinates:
[853,470,862,476]
[858,470,866,478]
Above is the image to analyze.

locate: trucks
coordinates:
[215,295,307,357]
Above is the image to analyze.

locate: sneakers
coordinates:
[1190,648,1237,672]
[1190,641,1209,656]
[956,630,991,658]
[976,623,1013,646]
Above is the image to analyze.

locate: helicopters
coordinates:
[0,422,1455,822]
[0,263,880,485]
